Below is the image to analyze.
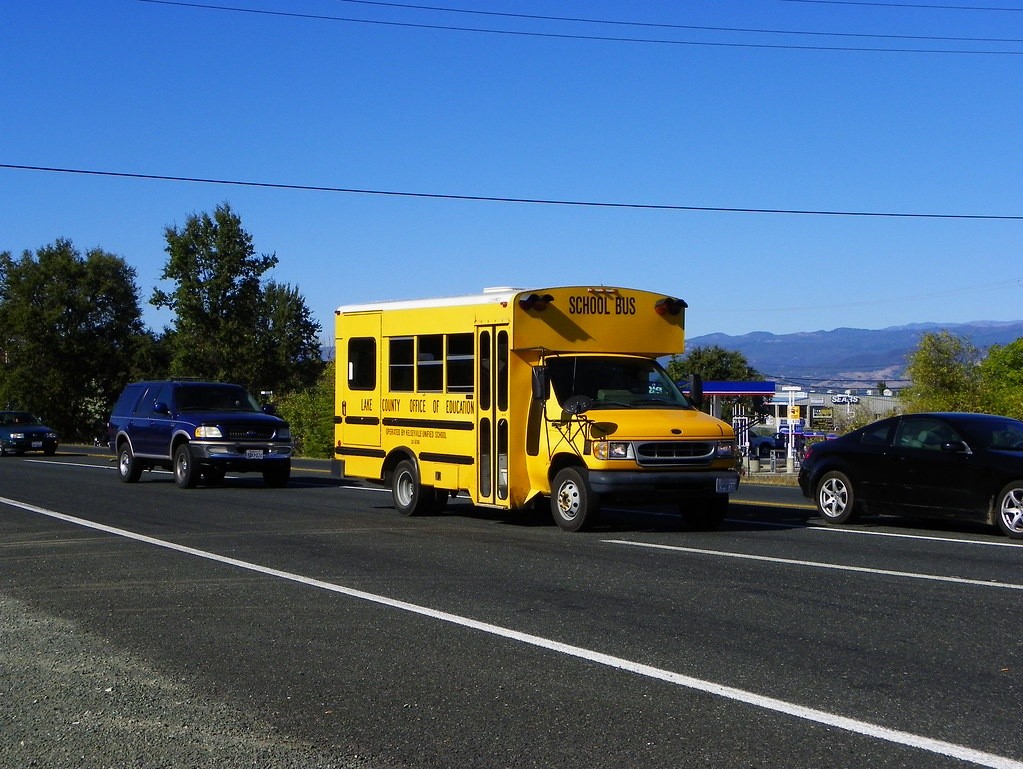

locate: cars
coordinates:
[736,429,837,457]
[798,412,1023,539]
[0,412,58,456]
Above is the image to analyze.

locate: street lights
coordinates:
[782,386,801,473]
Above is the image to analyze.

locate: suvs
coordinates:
[107,377,293,489]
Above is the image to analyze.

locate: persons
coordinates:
[923,429,983,452]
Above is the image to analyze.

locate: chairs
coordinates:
[912,431,943,450]
[6,419,14,424]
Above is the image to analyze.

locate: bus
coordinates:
[331,284,774,532]
